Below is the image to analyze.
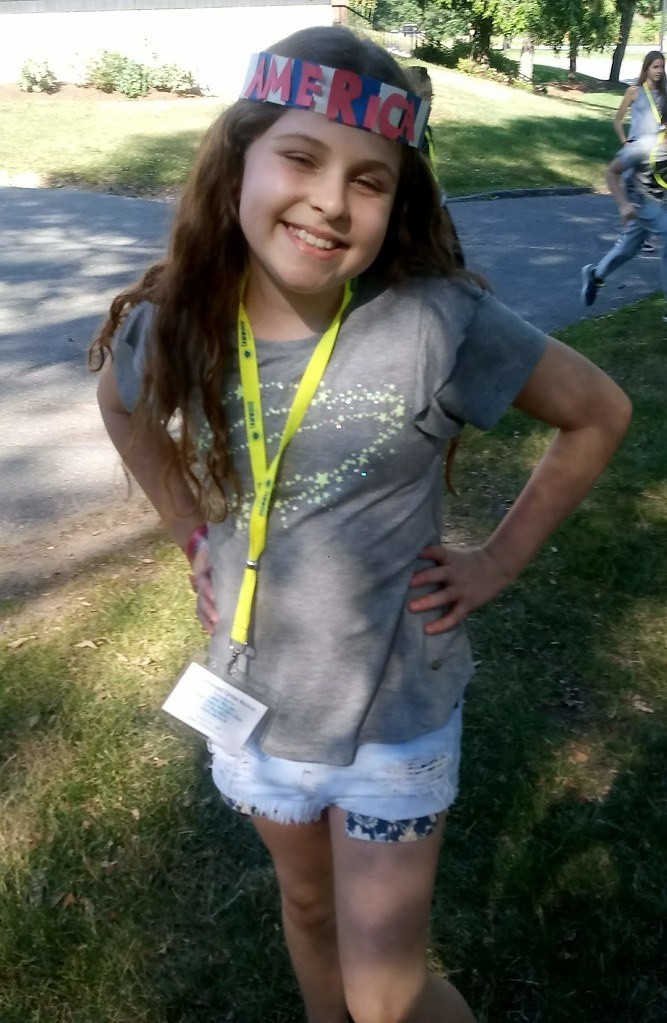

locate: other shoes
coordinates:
[640,241,654,252]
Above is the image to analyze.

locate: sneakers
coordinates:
[581,264,605,307]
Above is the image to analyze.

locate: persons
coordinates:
[581,89,667,320]
[87,24,634,1023]
[615,50,667,251]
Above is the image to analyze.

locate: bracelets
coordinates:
[186,524,208,567]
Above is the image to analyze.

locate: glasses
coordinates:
[420,91,434,100]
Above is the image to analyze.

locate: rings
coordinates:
[189,572,210,592]
